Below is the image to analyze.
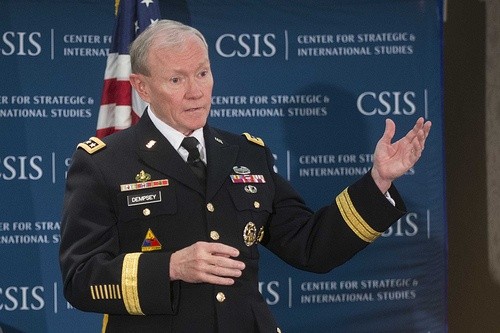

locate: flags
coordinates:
[95,1,165,142]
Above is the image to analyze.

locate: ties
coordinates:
[182,137,207,189]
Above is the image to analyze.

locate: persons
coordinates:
[60,18,436,331]
